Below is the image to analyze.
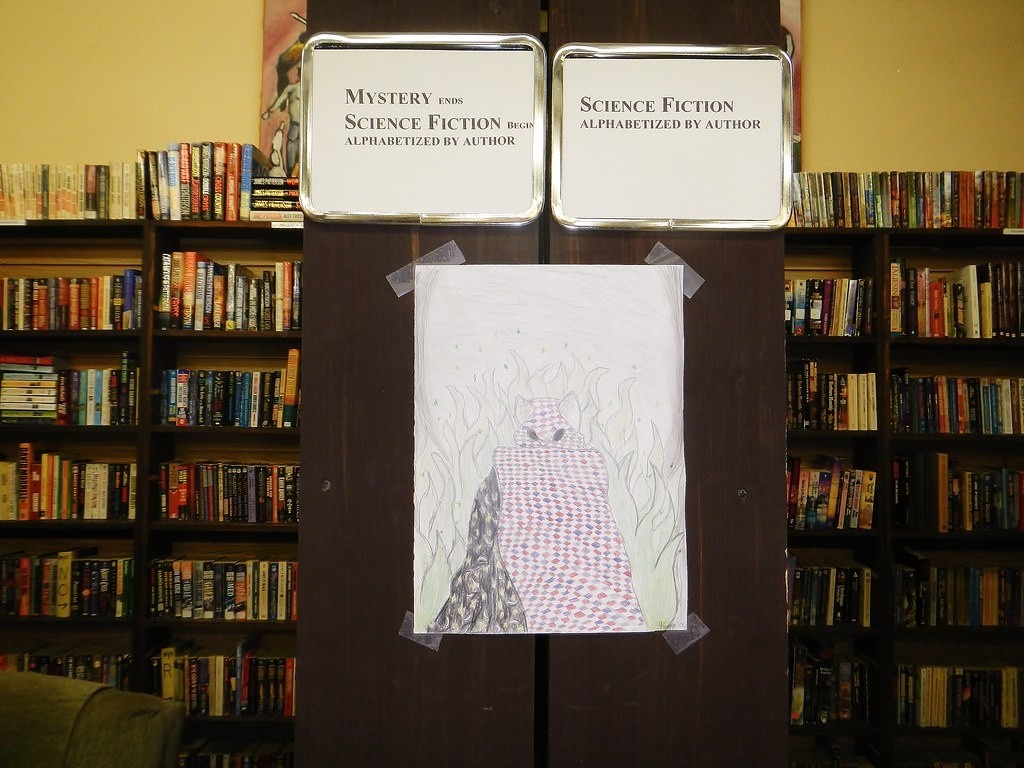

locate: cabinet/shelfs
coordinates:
[768,225,1024,768]
[292,0,790,766]
[0,219,303,726]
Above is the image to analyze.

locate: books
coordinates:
[787,170,1024,768]
[0,141,303,768]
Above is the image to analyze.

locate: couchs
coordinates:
[0,671,187,768]
